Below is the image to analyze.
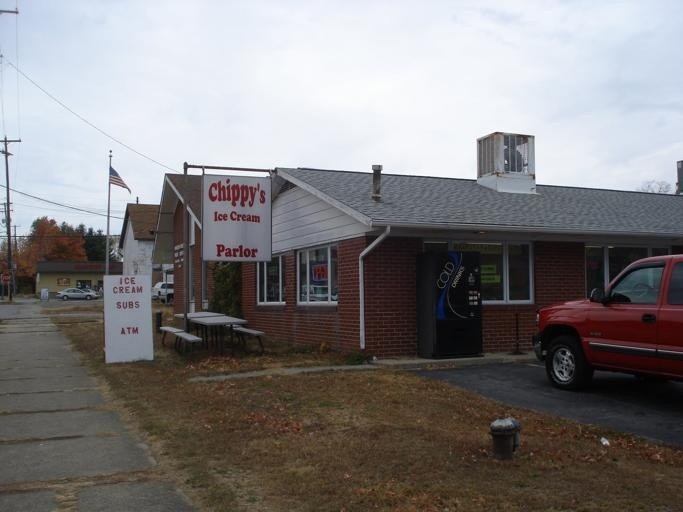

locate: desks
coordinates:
[175,311,248,354]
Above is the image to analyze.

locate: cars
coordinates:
[532,254,683,391]
[152,282,174,303]
[56,288,100,301]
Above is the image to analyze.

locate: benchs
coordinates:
[159,326,203,356]
[226,324,265,354]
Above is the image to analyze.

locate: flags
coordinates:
[110,165,131,195]
